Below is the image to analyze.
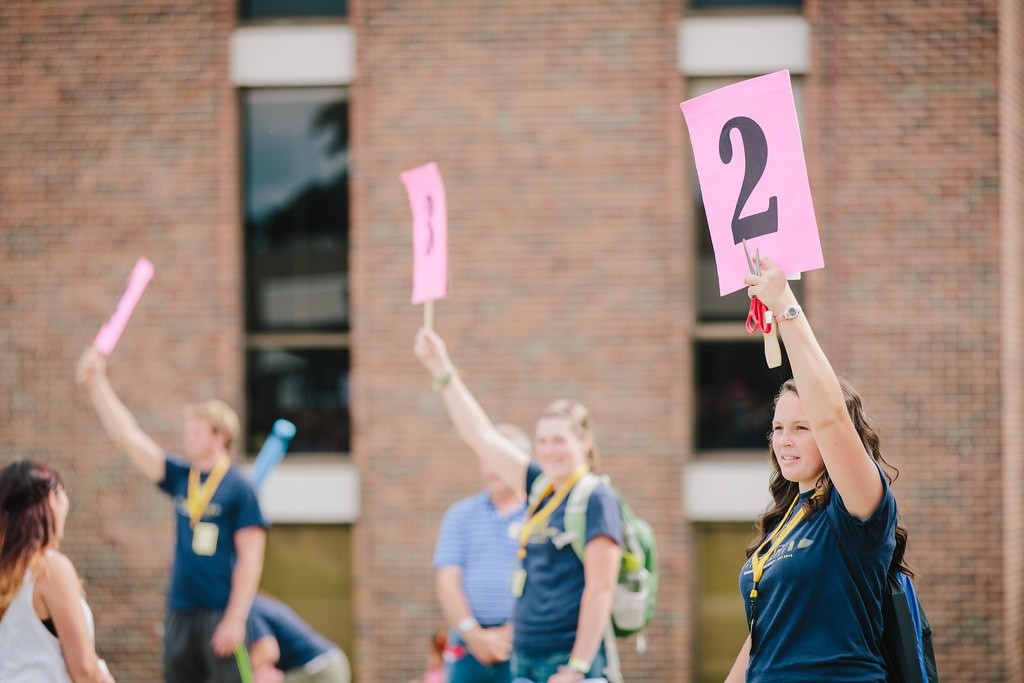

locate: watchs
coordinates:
[775,304,802,324]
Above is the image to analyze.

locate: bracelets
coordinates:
[567,657,592,673]
[433,366,457,390]
[456,618,479,634]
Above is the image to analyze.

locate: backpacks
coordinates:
[823,506,938,683]
[528,473,657,639]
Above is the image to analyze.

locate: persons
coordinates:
[246,596,352,683]
[432,422,535,683]
[75,343,272,683]
[724,256,920,683]
[0,458,117,682]
[413,328,624,683]
